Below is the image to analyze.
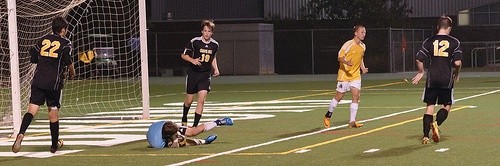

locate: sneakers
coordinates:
[50,139,64,153]
[12,132,24,153]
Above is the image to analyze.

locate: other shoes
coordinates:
[422,136,430,144]
[430,121,441,142]
[217,117,233,127]
[348,121,363,128]
[324,116,330,127]
[204,134,217,144]
[182,122,187,127]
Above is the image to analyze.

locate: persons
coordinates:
[13,17,76,154]
[412,16,462,144]
[324,25,368,128]
[147,118,234,148]
[181,20,220,128]
